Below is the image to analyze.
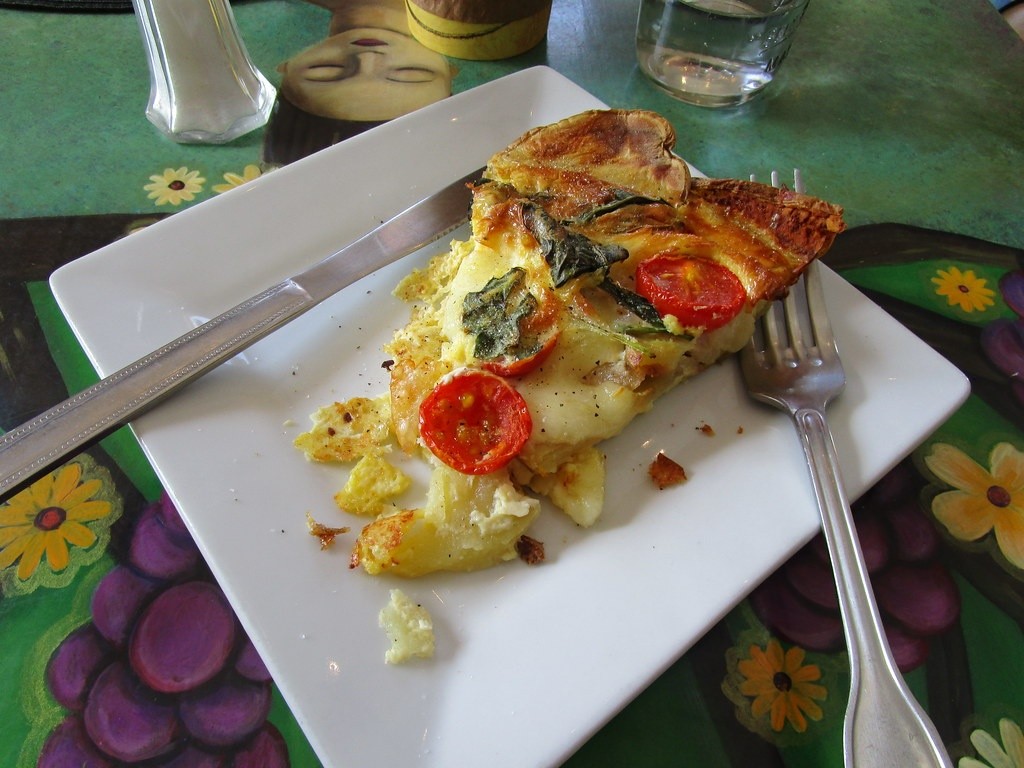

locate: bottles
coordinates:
[132,0,277,145]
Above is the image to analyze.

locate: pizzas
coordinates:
[294,106,845,581]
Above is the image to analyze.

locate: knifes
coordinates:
[0,165,489,505]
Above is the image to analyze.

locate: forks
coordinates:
[733,169,956,768]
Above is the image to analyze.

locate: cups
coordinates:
[634,0,810,108]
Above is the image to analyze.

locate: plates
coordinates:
[49,65,971,768]
